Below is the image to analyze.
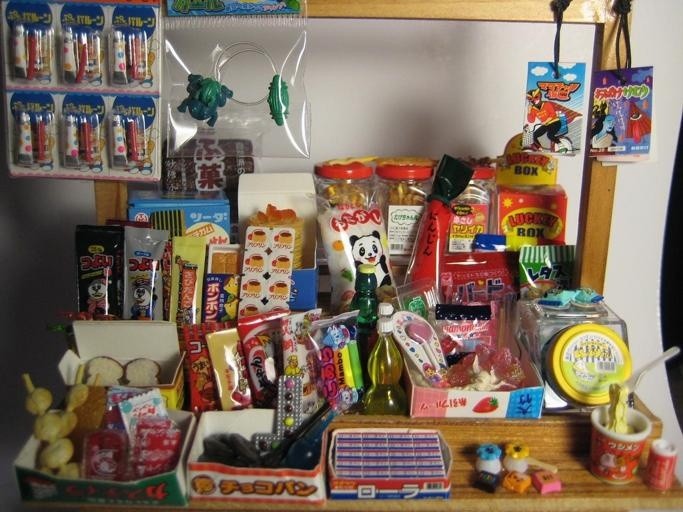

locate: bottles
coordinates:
[351,263,408,416]
[514,296,631,408]
[315,158,497,264]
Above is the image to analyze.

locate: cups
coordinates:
[589,404,652,486]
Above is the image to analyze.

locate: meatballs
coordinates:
[26,382,82,481]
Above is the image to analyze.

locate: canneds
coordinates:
[312,161,496,266]
